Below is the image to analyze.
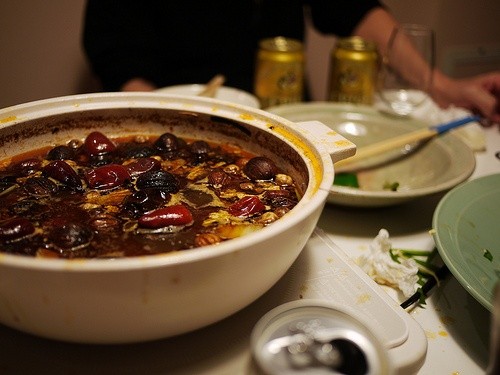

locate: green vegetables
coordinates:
[380,181,493,307]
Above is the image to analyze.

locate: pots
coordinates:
[0,92,357,344]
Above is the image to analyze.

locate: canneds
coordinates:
[326,36,380,105]
[246,300,394,375]
[256,36,305,111]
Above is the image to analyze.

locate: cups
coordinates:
[376,23,434,111]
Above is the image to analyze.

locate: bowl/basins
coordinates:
[264,101,476,207]
[432,172,500,314]
[155,84,259,109]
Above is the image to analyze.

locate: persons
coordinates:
[80,0,500,123]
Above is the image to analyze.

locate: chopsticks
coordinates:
[335,115,480,166]
